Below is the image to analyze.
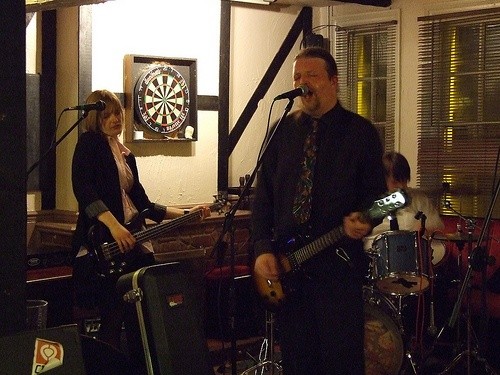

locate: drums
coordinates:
[360,249,381,308]
[370,228,431,298]
[362,301,408,375]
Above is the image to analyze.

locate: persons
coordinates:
[362,152,446,354]
[253,47,388,375]
[72,92,212,322]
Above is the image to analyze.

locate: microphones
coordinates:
[68,100,106,112]
[273,85,309,100]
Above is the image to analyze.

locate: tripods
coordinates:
[241,311,283,375]
[439,181,500,375]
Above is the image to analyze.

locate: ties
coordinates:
[292,118,317,224]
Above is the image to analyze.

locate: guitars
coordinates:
[254,186,411,320]
[87,203,210,290]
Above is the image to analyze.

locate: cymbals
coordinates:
[433,233,490,244]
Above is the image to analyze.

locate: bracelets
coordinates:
[183,208,190,215]
